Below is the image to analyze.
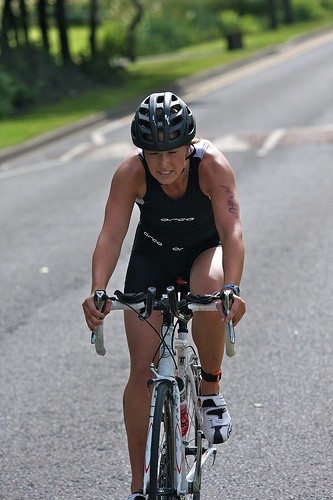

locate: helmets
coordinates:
[131,92,196,151]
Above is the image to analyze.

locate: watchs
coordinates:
[220,283,240,297]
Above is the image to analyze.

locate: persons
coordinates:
[81,92,246,500]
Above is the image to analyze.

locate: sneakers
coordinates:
[127,494,148,500]
[197,393,232,444]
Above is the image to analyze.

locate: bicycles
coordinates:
[91,277,236,500]
